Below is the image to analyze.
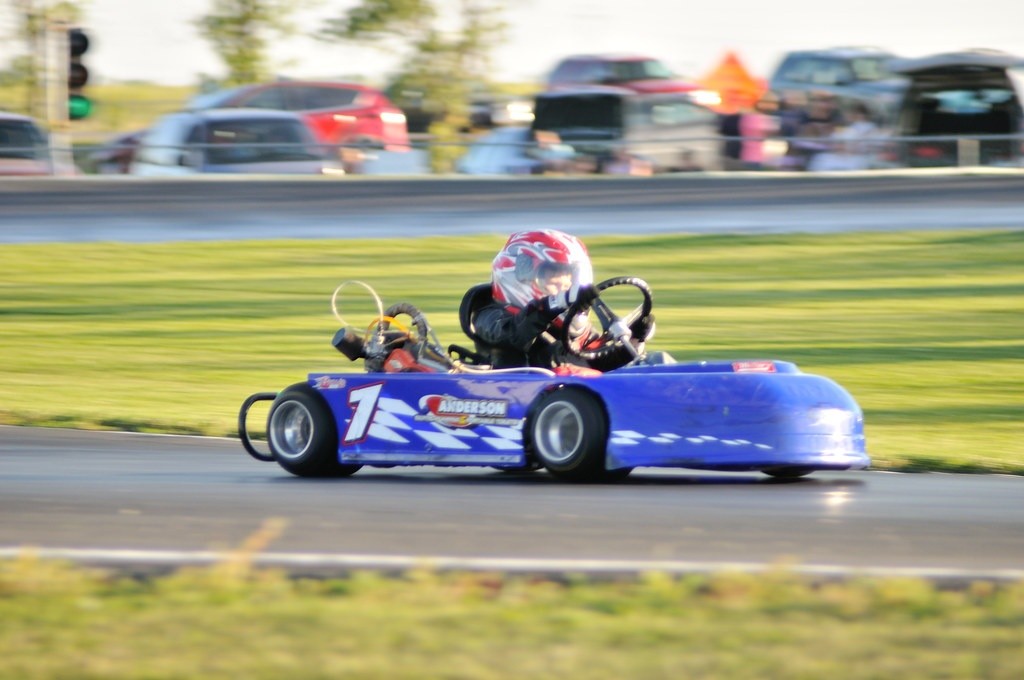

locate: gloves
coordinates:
[548,285,591,315]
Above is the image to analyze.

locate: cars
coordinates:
[0,112,84,176]
[129,104,346,178]
[411,45,1024,174]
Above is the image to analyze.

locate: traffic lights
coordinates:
[66,26,93,123]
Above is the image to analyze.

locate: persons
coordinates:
[471,229,677,378]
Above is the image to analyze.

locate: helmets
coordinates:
[491,230,594,336]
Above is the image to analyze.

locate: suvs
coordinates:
[86,79,409,181]
